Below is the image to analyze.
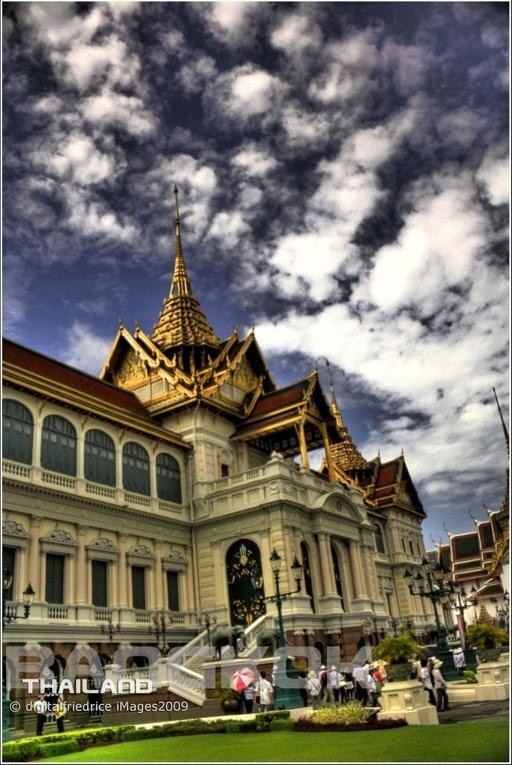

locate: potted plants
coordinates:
[215,687,243,714]
[465,624,509,662]
[370,633,428,682]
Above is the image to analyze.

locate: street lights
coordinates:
[492,590,510,625]
[146,614,163,650]
[3,573,37,696]
[196,610,220,648]
[152,608,174,656]
[438,583,479,650]
[403,554,486,649]
[247,545,308,710]
[387,615,399,637]
[99,617,122,642]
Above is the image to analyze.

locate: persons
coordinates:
[242,683,256,715]
[54,696,67,733]
[299,658,387,707]
[34,694,48,736]
[212,628,245,662]
[255,670,274,714]
[411,656,450,713]
[445,625,467,676]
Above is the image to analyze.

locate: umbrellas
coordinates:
[230,667,255,692]
